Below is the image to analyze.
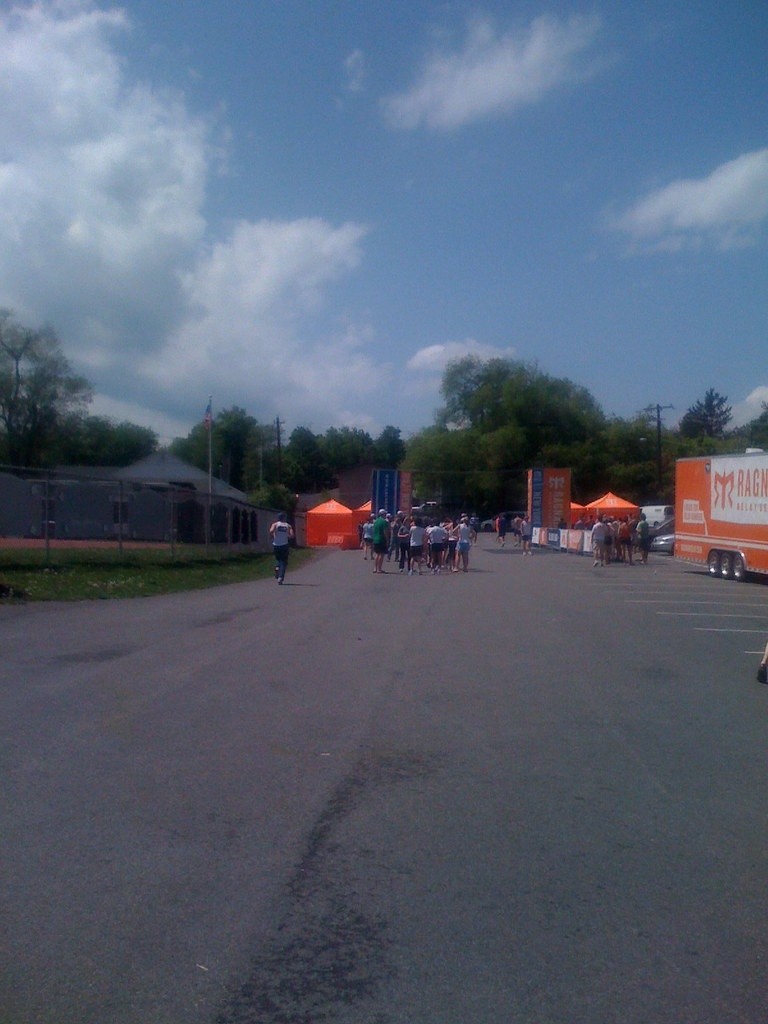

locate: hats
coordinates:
[379,509,388,515]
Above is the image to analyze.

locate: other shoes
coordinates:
[278,580,282,586]
[601,563,603,566]
[593,560,598,567]
[275,569,280,578]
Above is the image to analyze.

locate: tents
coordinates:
[571,492,639,524]
[306,498,371,546]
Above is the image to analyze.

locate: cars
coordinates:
[412,501,437,514]
[634,504,674,556]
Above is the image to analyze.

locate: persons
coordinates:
[496,513,506,547]
[512,513,534,556]
[358,509,479,576]
[269,512,294,585]
[760,642,768,667]
[574,513,648,567]
[558,518,566,529]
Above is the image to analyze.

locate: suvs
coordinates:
[480,511,528,532]
